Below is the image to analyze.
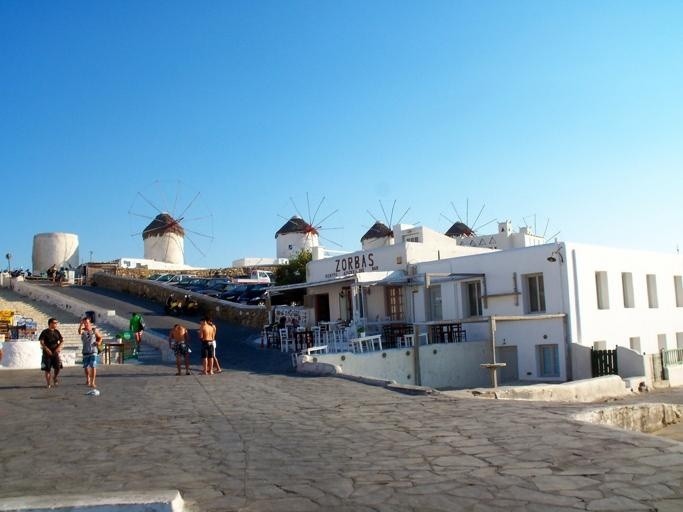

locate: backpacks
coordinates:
[92,326,104,354]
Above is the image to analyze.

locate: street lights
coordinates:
[5,250,12,289]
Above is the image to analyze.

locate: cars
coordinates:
[145,269,304,306]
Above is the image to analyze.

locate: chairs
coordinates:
[259,319,466,369]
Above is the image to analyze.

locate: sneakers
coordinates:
[175,370,222,376]
[46,377,96,388]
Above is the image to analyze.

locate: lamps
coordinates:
[545,244,565,264]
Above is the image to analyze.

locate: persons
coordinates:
[197,319,215,375]
[200,317,222,374]
[169,323,192,376]
[128,311,145,358]
[78,317,103,389]
[38,318,64,389]
